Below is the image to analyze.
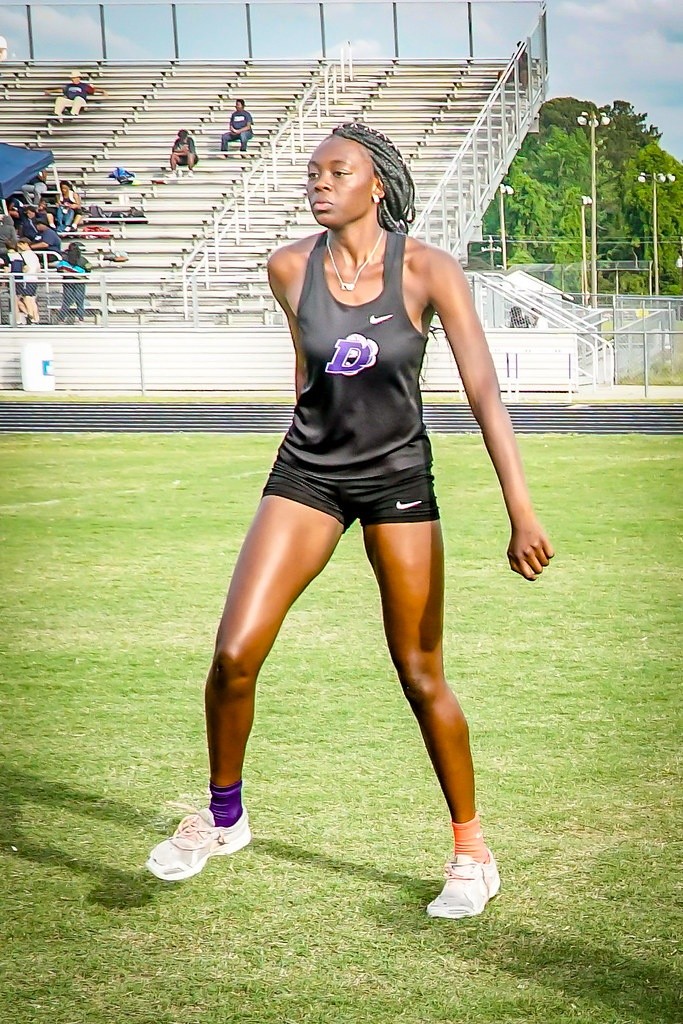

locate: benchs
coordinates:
[0,59,541,324]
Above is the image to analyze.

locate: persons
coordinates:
[497,41,530,100]
[506,303,539,328]
[44,70,108,124]
[0,36,8,62]
[56,243,92,322]
[216,99,254,160]
[0,166,82,264]
[16,237,41,324]
[140,123,556,920]
[165,129,199,178]
[0,239,32,324]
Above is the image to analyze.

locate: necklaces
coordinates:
[326,227,383,292]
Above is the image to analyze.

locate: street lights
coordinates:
[498,184,514,269]
[580,195,591,306]
[578,110,611,308]
[638,171,675,295]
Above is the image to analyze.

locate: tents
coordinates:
[0,143,64,216]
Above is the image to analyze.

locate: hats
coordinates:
[69,70,82,78]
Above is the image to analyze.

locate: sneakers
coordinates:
[146,807,251,880]
[426,848,501,918]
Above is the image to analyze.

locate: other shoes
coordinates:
[26,314,33,324]
[188,170,194,177]
[241,151,251,158]
[219,154,228,159]
[16,323,23,325]
[166,171,177,177]
